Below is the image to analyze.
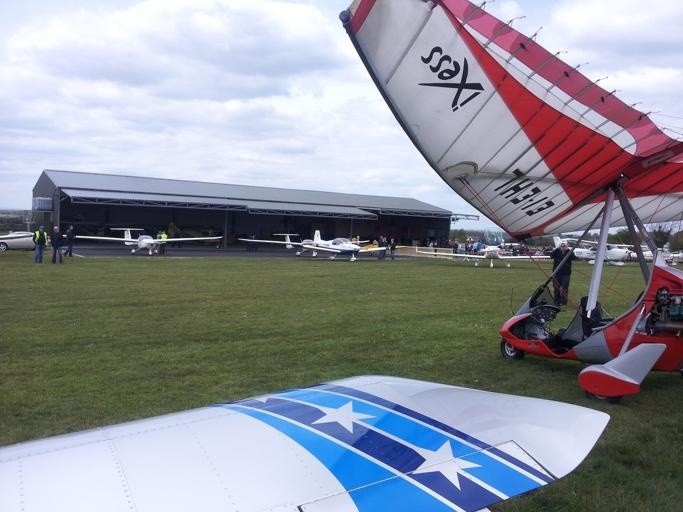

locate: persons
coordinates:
[63,225,74,257]
[49,225,64,264]
[509,244,529,256]
[373,235,395,259]
[32,224,47,263]
[550,239,576,309]
[430,237,477,259]
[157,232,257,255]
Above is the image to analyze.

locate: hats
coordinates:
[559,239,568,250]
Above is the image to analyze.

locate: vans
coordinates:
[198,229,224,246]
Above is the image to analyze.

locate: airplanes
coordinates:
[54,226,225,257]
[336,1,683,403]
[237,229,406,262]
[413,226,551,268]
[0,370,617,511]
[550,232,647,267]
[351,237,402,257]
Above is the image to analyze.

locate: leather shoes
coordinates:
[554,303,565,312]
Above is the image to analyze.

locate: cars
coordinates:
[0,230,50,251]
[630,243,683,264]
